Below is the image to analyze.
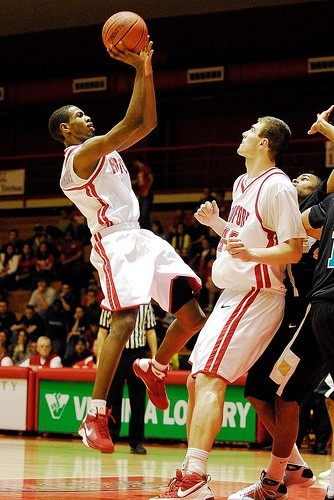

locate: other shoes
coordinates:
[130,445,147,454]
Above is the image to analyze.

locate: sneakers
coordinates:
[148,468,215,500]
[283,463,316,488]
[226,469,289,500]
[77,407,115,453]
[133,358,170,410]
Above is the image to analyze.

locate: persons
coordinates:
[50,35,206,452]
[152,188,230,350]
[149,117,309,499]
[227,105,334,499]
[0,201,105,367]
[133,154,154,227]
[95,302,157,454]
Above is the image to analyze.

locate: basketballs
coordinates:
[102,11,148,56]
